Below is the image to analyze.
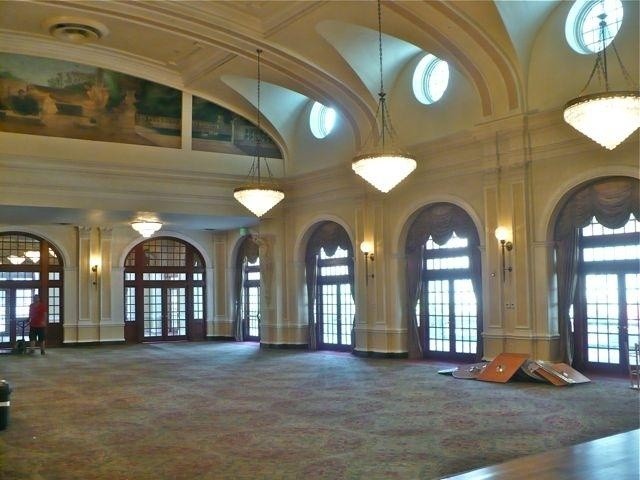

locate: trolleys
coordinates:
[13,319,34,355]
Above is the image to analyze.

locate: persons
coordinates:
[97,81,109,112]
[25,294,48,355]
[15,88,38,117]
[83,83,109,104]
[25,83,59,115]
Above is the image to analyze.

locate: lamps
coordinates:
[234,47,285,219]
[348,0,417,194]
[494,225,513,284]
[359,240,374,288]
[562,0,640,152]
